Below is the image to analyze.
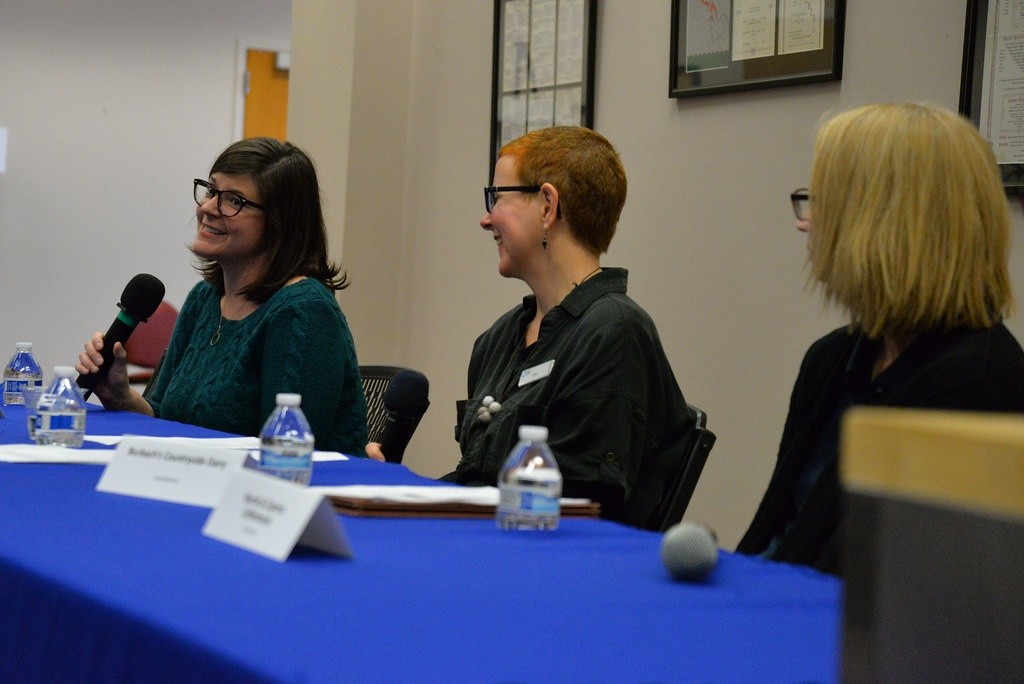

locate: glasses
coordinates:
[193,178,268,217]
[484,185,562,219]
[791,188,816,221]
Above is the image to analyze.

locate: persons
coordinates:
[75,136,367,456]
[364,127,690,530]
[733,102,1024,578]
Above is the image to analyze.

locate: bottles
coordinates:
[33,366,86,448]
[3,341,43,406]
[260,393,313,486]
[495,425,563,532]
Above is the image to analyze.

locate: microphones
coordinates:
[659,521,719,579]
[75,273,166,403]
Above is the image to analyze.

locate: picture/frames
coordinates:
[669,0,848,97]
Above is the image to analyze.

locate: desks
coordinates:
[0,382,847,684]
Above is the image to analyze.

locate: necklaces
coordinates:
[210,298,246,346]
[579,267,600,284]
